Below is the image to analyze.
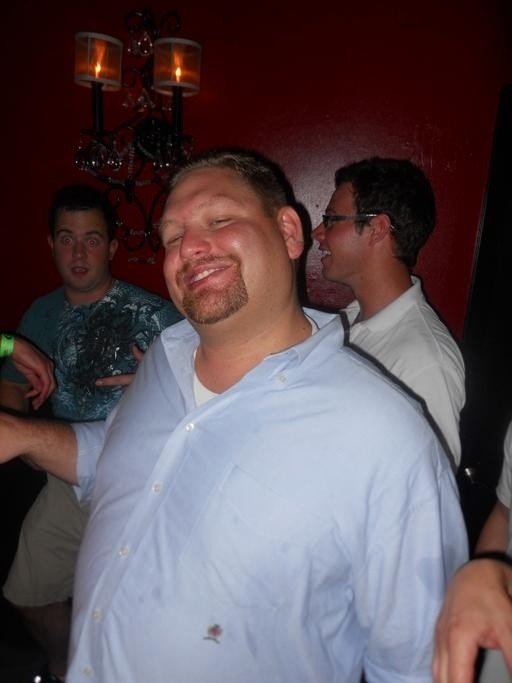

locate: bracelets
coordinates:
[0,332,16,361]
[468,549,510,567]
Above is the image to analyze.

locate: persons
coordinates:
[306,155,469,478]
[430,416,510,682]
[0,148,469,682]
[0,332,61,415]
[1,181,189,681]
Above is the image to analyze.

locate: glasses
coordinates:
[322,214,377,229]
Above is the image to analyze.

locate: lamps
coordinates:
[73,0,202,253]
